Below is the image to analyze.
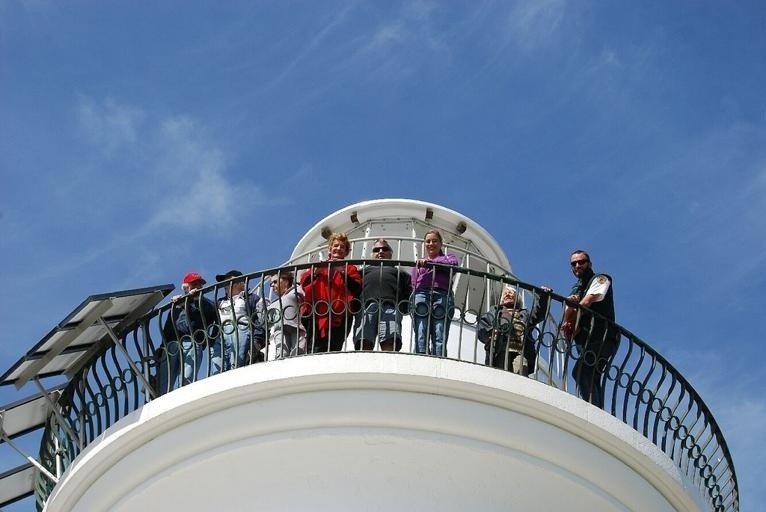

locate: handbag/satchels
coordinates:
[340,270,344,275]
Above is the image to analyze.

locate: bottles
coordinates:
[182,273,207,286]
[215,270,244,281]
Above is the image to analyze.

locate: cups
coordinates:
[570,259,588,267]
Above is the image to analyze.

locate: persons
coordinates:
[211,270,264,374]
[477,284,554,378]
[353,239,411,351]
[156,272,216,395]
[300,235,362,354]
[256,272,308,361]
[560,249,617,409]
[411,230,458,357]
